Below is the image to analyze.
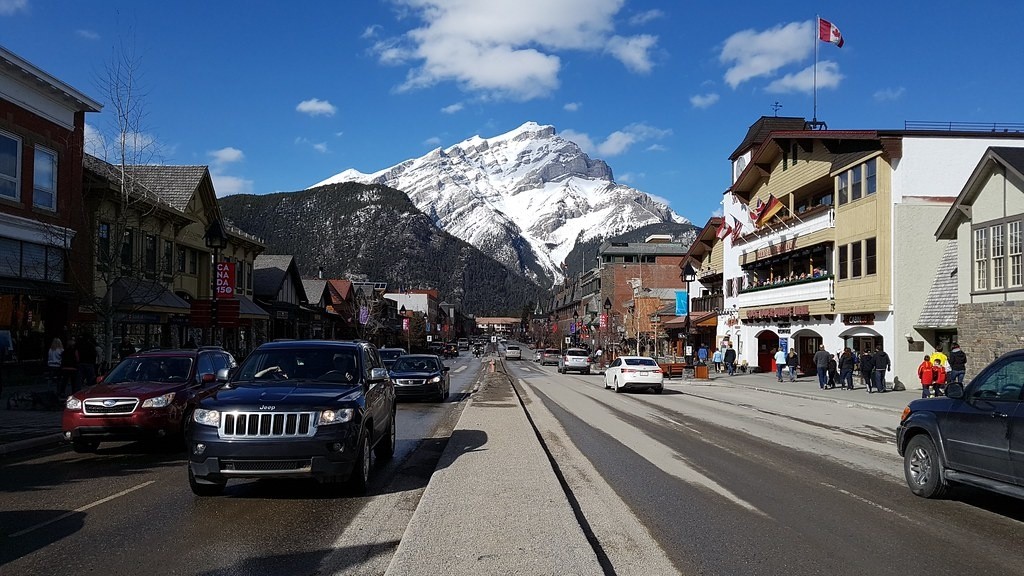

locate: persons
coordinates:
[860,348,875,393]
[871,344,891,393]
[813,344,837,388]
[839,347,860,391]
[747,266,823,288]
[254,356,288,379]
[424,360,434,370]
[595,348,602,359]
[319,350,354,381]
[932,359,947,397]
[381,344,385,349]
[929,345,947,367]
[119,334,136,362]
[724,344,736,375]
[182,335,198,349]
[787,348,800,382]
[773,347,787,382]
[47,332,103,399]
[918,355,934,399]
[693,343,709,364]
[947,341,967,384]
[711,348,722,373]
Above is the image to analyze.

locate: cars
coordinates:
[897,349,1024,500]
[604,356,664,394]
[429,342,459,357]
[532,348,561,366]
[388,355,450,403]
[497,334,510,344]
[469,335,490,353]
[378,349,406,371]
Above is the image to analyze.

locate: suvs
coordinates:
[558,348,590,375]
[186,339,397,494]
[61,346,237,452]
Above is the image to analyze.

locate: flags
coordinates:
[715,218,733,242]
[729,217,744,249]
[746,206,757,227]
[755,198,775,224]
[755,194,784,229]
[817,17,844,47]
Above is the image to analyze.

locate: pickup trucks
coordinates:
[457,338,469,350]
[503,344,522,360]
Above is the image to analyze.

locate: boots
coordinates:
[866,383,871,393]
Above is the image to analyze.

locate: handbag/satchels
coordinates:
[945,360,952,372]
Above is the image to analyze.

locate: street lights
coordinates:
[572,310,579,347]
[679,260,698,380]
[603,298,613,359]
[201,217,228,347]
[399,304,406,347]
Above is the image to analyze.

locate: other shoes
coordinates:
[778,379,783,382]
[719,369,721,374]
[847,386,853,391]
[823,384,827,390]
